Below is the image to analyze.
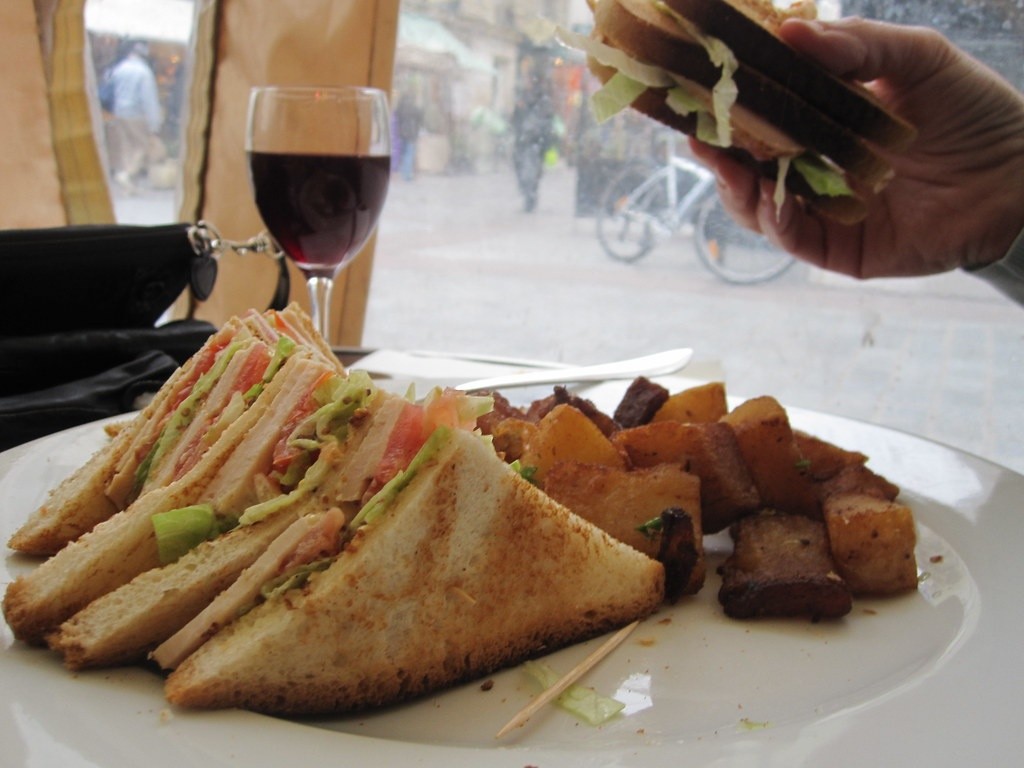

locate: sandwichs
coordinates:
[568,0,921,227]
[5,302,669,721]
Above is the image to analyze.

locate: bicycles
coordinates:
[595,122,799,284]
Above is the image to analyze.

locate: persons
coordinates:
[686,18,1024,303]
[512,70,555,210]
[111,41,162,199]
[395,83,424,180]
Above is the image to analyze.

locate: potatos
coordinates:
[481,380,920,599]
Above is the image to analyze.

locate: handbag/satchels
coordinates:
[1,222,219,448]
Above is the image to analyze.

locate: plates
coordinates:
[1,380,1024,768]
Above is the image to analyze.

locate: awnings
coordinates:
[394,17,487,72]
[85,1,193,39]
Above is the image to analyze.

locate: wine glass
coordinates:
[245,85,392,343]
